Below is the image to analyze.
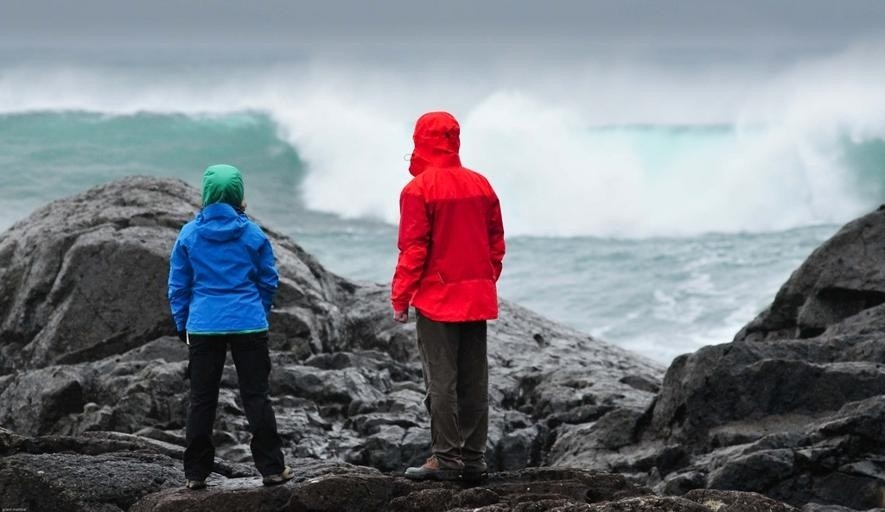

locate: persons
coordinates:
[165,164,292,488]
[390,111,506,475]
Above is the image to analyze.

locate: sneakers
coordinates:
[404,454,460,481]
[263,465,295,484]
[464,468,488,482]
[185,478,204,490]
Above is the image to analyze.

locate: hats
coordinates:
[201,164,244,208]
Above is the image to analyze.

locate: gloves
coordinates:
[177,329,186,343]
